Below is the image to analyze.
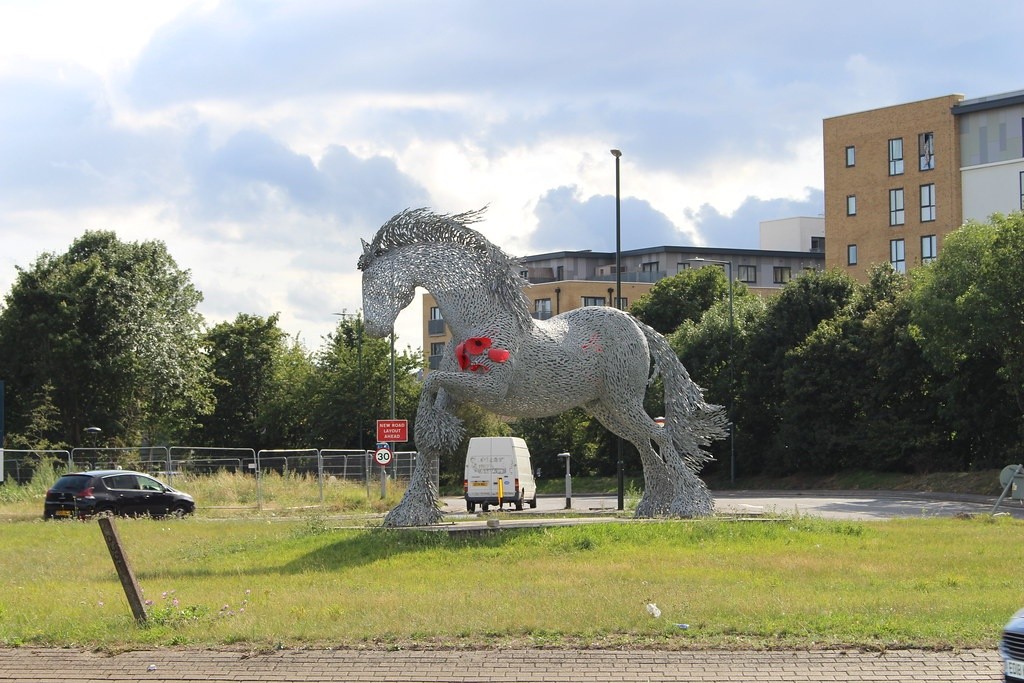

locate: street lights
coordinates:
[609,146,625,509]
[685,256,737,485]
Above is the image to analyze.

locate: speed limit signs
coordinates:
[375,448,393,466]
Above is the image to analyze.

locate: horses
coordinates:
[357,203,734,527]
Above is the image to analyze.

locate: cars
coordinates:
[44,469,194,522]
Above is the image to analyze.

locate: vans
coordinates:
[462,435,537,511]
